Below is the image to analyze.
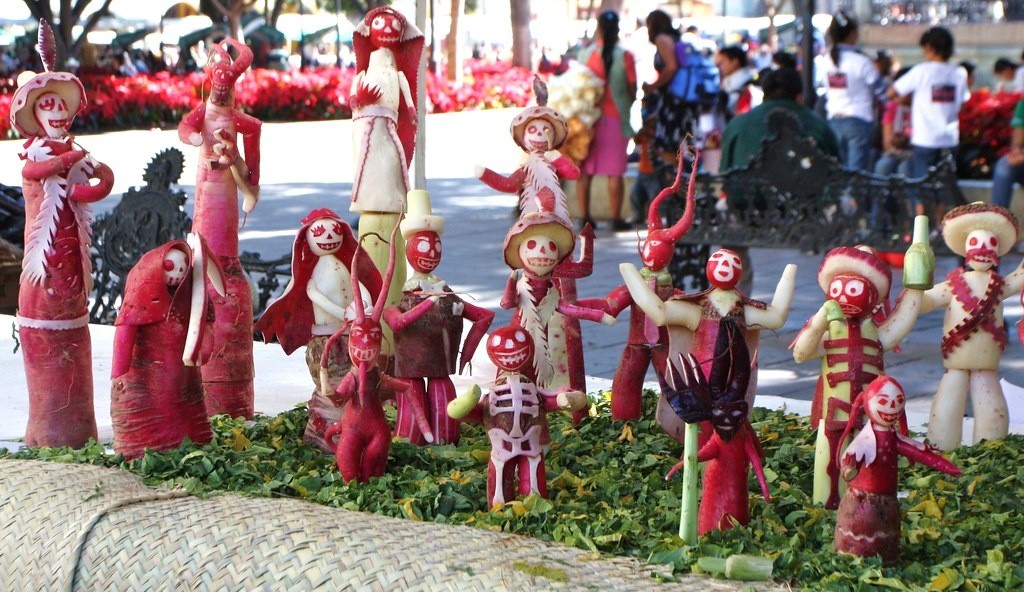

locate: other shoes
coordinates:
[579,215,597,231]
[608,216,632,230]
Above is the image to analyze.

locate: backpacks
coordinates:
[670,40,720,103]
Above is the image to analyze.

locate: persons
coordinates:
[577,10,1024,228]
[10,6,496,479]
[446,74,1024,560]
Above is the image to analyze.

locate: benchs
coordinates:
[647,109,1010,347]
